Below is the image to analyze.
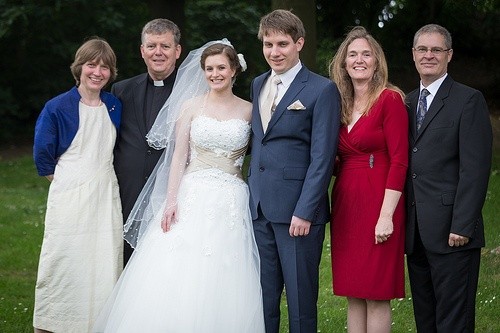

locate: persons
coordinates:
[330,26,409,333]
[92,38,265,333]
[33,39,124,333]
[247,9,342,333]
[111,19,181,270]
[405,24,493,333]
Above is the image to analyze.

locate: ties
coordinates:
[261,76,282,135]
[416,89,431,137]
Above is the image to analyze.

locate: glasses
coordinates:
[414,47,451,54]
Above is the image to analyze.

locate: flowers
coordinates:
[237,54,247,72]
